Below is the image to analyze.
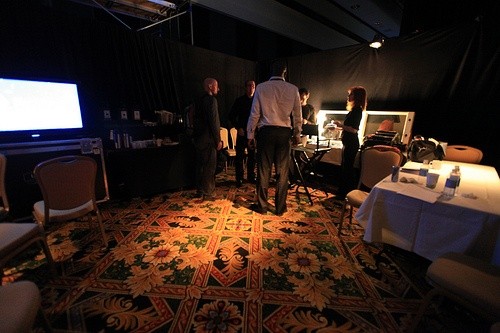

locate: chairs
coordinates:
[0,280,53,333]
[31,154,110,253]
[443,145,484,164]
[423,257,500,333]
[219,127,236,173]
[336,144,404,238]
[230,127,249,169]
[0,222,57,284]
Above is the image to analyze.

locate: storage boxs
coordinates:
[0,138,110,223]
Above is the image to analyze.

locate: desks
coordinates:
[304,139,345,165]
[353,159,500,279]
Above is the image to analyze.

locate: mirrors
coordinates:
[357,110,415,152]
[307,109,363,149]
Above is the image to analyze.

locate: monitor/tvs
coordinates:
[0,74,89,142]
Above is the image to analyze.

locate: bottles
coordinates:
[418,160,429,185]
[114,128,129,149]
[443,165,461,198]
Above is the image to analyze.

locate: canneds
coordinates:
[391,165,399,182]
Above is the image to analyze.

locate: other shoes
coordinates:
[195,192,204,197]
[276,205,286,215]
[247,177,257,182]
[257,204,267,214]
[237,179,242,186]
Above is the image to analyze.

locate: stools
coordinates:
[289,124,331,205]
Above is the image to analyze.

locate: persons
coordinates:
[230,80,258,187]
[191,78,223,199]
[332,86,367,210]
[289,86,315,189]
[246,61,303,216]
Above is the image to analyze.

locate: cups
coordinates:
[302,136,307,147]
[429,160,442,170]
[426,172,440,189]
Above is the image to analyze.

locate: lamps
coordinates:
[368,34,384,48]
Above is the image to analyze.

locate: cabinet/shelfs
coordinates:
[100,119,195,202]
